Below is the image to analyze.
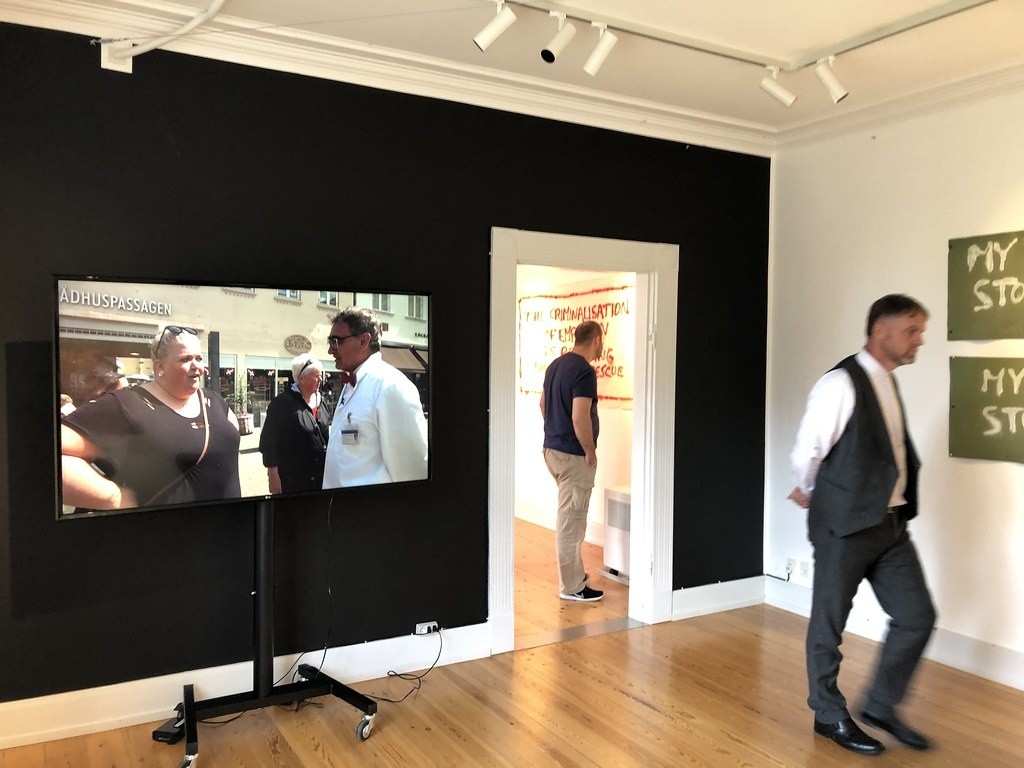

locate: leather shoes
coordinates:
[813,714,886,755]
[860,709,929,751]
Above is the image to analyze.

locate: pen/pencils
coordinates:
[346,412,352,424]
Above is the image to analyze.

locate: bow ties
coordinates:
[339,372,358,388]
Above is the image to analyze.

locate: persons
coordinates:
[102,372,130,393]
[540,320,604,601]
[258,353,329,494]
[60,325,241,510]
[787,294,937,756]
[322,308,427,490]
[61,393,73,419]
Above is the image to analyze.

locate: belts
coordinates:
[886,507,899,514]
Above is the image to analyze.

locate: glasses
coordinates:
[327,333,358,350]
[299,359,313,376]
[155,326,199,358]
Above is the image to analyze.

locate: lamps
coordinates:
[814,54,850,104]
[473,0,517,54]
[540,9,576,65]
[582,21,618,77]
[759,65,798,109]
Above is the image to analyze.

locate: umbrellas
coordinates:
[104,356,123,368]
[126,374,150,383]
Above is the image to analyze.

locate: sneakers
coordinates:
[583,573,588,584]
[560,586,603,601]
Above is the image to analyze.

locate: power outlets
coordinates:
[799,561,810,577]
[787,558,797,574]
[416,621,438,635]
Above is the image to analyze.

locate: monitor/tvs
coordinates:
[51,273,433,521]
